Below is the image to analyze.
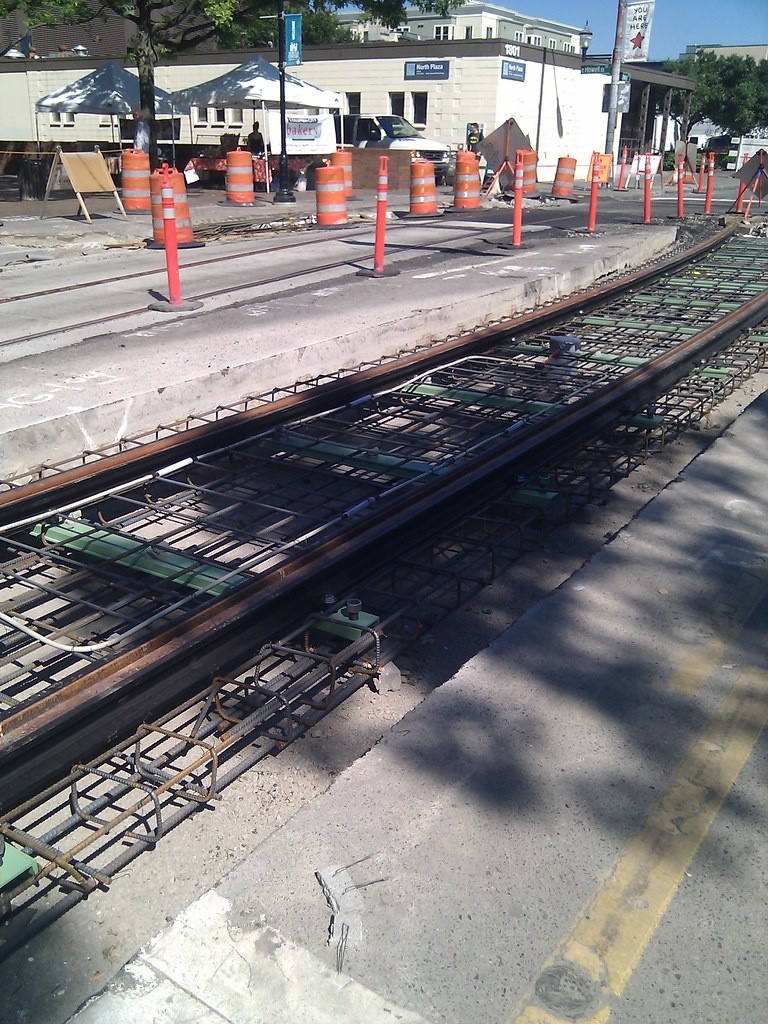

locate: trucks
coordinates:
[686,134,711,150]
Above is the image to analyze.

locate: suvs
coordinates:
[333,113,453,187]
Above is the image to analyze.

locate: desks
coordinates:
[190,155,300,192]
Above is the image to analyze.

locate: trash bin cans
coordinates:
[16,159,46,201]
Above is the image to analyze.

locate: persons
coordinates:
[131,108,152,153]
[248,121,263,156]
[467,123,485,164]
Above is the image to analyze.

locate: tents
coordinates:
[36,60,345,194]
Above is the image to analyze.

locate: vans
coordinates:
[701,135,737,160]
